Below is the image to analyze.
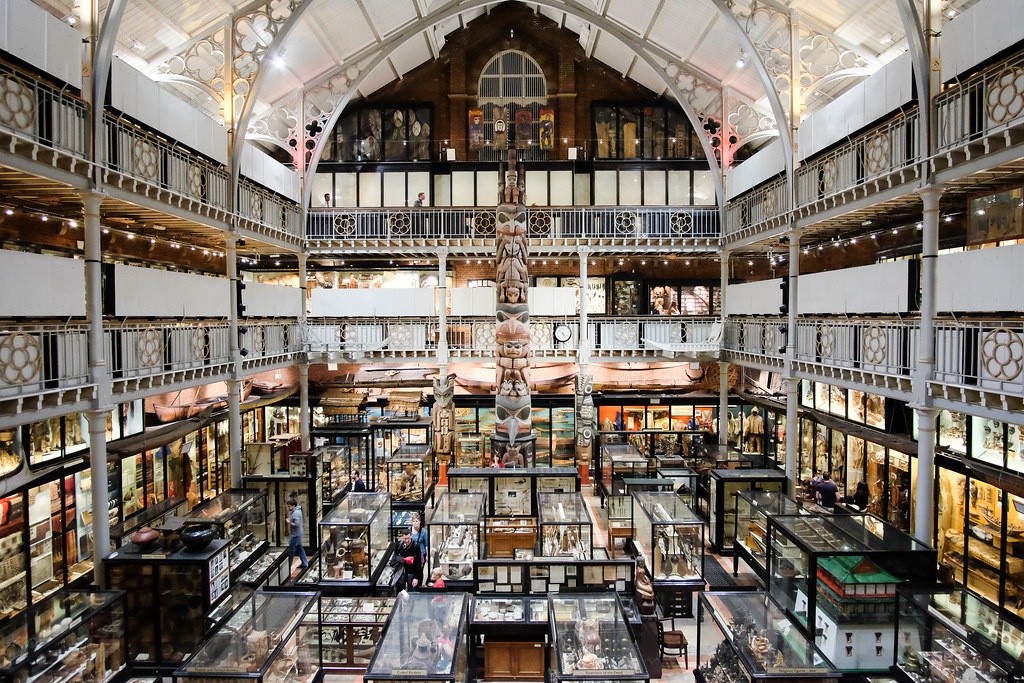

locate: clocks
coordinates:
[554,324,572,342]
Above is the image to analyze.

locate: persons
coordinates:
[672,420,689,430]
[653,298,679,316]
[488,454,505,468]
[320,193,332,207]
[728,405,764,453]
[285,500,308,569]
[350,470,365,492]
[414,192,426,207]
[853,481,869,513]
[815,471,841,507]
[332,458,348,474]
[398,514,427,590]
[600,417,614,432]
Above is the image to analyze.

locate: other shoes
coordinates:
[297,564,307,568]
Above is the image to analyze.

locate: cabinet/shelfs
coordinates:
[0,406,268,677]
[888,580,1024,683]
[479,516,537,559]
[295,596,396,675]
[455,435,485,469]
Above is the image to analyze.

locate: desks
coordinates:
[608,519,700,559]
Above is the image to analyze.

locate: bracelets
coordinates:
[289,521,291,524]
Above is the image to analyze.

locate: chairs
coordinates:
[658,617,688,670]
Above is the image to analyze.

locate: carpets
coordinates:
[698,554,737,586]
